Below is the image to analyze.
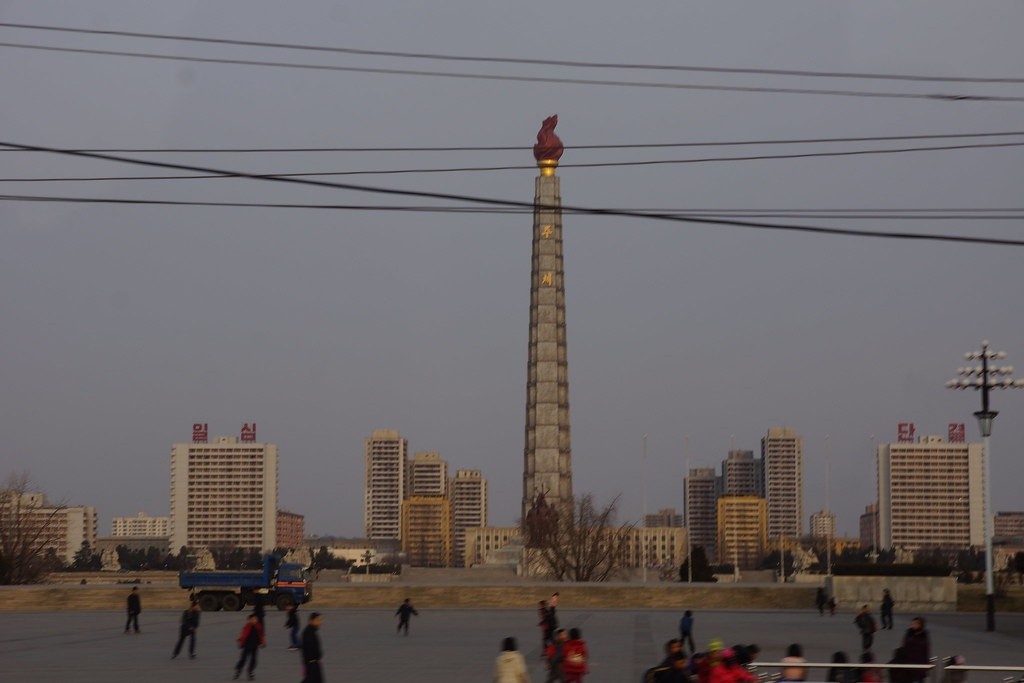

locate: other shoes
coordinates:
[235,671,240,680]
[248,672,256,682]
[169,654,176,659]
[285,646,299,650]
[188,654,196,659]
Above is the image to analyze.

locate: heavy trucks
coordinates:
[178,553,313,613]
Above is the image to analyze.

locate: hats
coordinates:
[709,638,726,651]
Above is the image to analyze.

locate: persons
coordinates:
[300,612,324,683]
[945,655,968,683]
[124,587,141,633]
[395,598,419,635]
[231,613,265,682]
[491,637,532,683]
[283,604,301,652]
[879,588,895,631]
[640,637,761,683]
[815,587,836,616]
[825,651,889,683]
[537,593,590,683]
[678,609,696,654]
[885,616,932,683]
[171,601,202,661]
[854,604,878,652]
[781,644,810,682]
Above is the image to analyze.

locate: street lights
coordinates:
[944,339,1024,632]
